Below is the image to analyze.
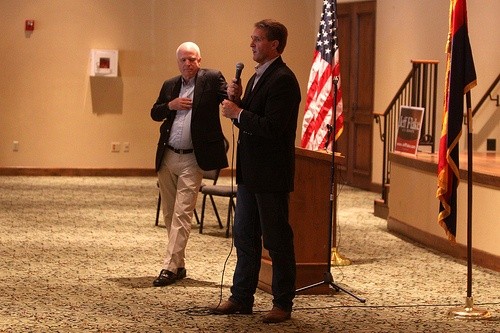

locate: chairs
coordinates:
[155,167,238,239]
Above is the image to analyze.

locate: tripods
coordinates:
[296,76,366,303]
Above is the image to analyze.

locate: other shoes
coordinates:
[207,297,252,314]
[152,269,177,287]
[263,305,291,322]
[176,268,186,278]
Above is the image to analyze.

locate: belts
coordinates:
[166,144,193,154]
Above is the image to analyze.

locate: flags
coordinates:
[299,0,344,153]
[436,0,478,248]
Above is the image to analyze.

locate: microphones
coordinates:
[232,62,244,99]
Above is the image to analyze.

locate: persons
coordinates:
[150,41,229,286]
[208,19,302,323]
